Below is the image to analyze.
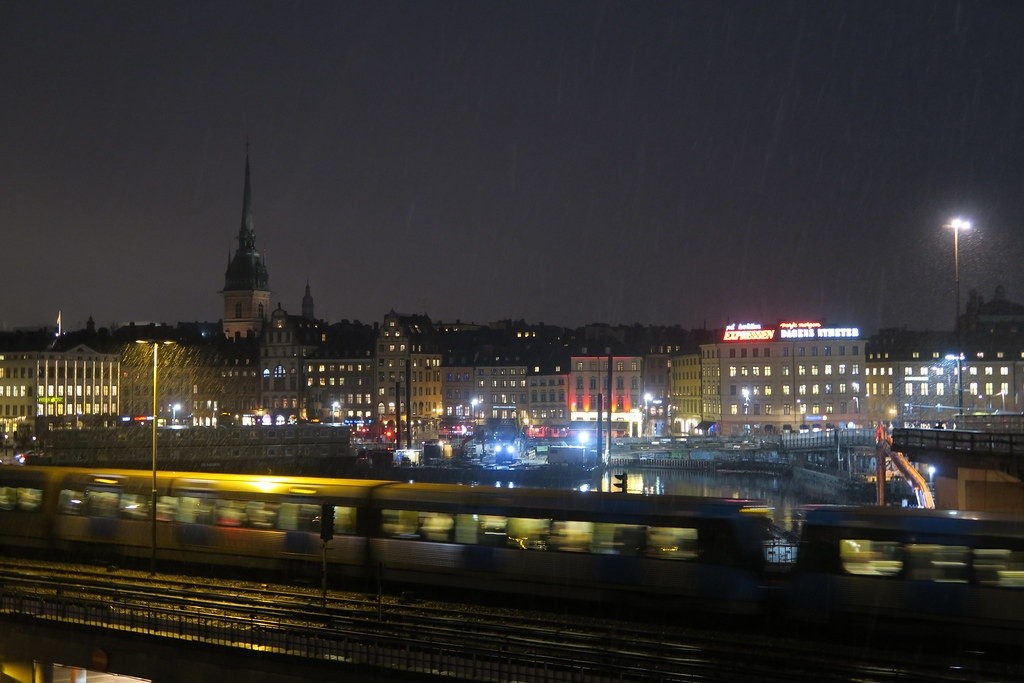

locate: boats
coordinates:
[788,422,936,507]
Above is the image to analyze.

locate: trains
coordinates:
[0,462,1024,660]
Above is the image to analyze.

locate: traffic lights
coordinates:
[612,472,629,492]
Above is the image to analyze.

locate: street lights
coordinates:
[939,218,974,425]
[135,337,179,575]
